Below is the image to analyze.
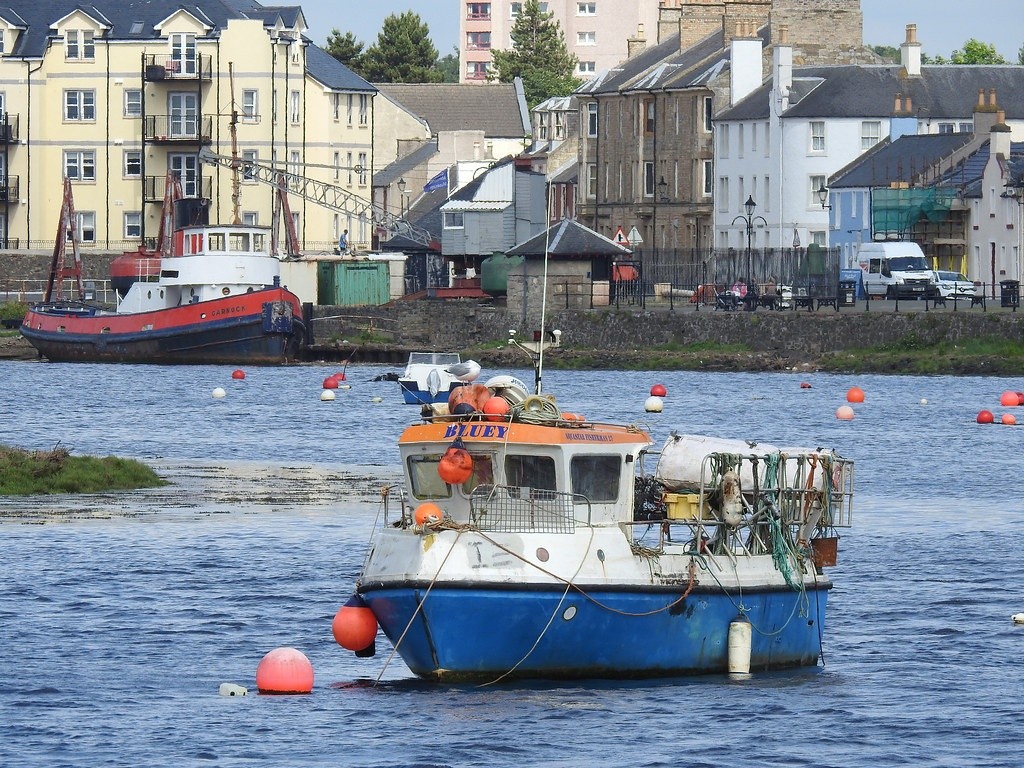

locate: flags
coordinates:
[423,169,448,192]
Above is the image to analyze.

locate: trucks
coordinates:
[852,242,936,300]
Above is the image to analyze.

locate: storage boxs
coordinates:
[661,493,720,520]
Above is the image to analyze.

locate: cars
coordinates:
[931,271,974,300]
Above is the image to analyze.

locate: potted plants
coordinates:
[0,186,5,199]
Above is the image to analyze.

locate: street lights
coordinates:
[404,189,414,221]
[731,194,767,286]
[398,175,406,220]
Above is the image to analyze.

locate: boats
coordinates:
[357,178,854,686]
[397,345,472,405]
[19,62,306,365]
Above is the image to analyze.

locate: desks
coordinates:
[816,298,836,311]
[968,296,986,308]
[794,298,816,311]
[930,297,947,308]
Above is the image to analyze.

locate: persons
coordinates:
[339,229,348,251]
[731,277,760,311]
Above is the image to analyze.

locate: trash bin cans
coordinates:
[999,279,1020,312]
[837,279,857,307]
